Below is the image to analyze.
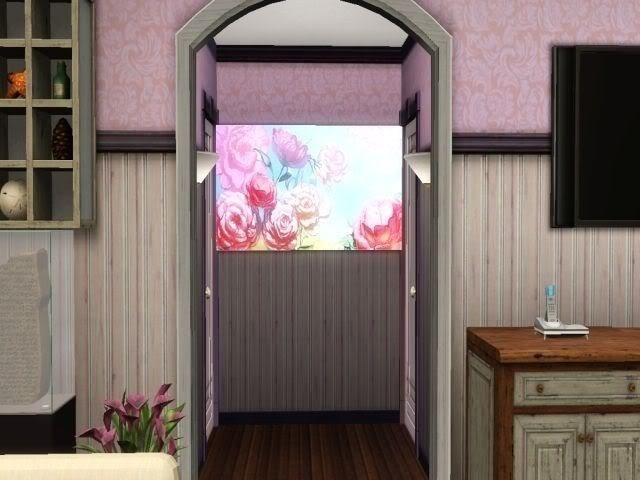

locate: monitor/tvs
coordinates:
[551,43,640,227]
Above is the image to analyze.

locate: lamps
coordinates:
[196,151,221,187]
[404,152,431,186]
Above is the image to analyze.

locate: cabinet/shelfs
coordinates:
[466,326,639,479]
[0,0,96,231]
[1,230,76,455]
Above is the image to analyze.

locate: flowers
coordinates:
[75,383,191,456]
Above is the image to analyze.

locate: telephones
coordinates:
[534,279,589,340]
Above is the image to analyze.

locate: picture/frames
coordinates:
[215,124,402,251]
